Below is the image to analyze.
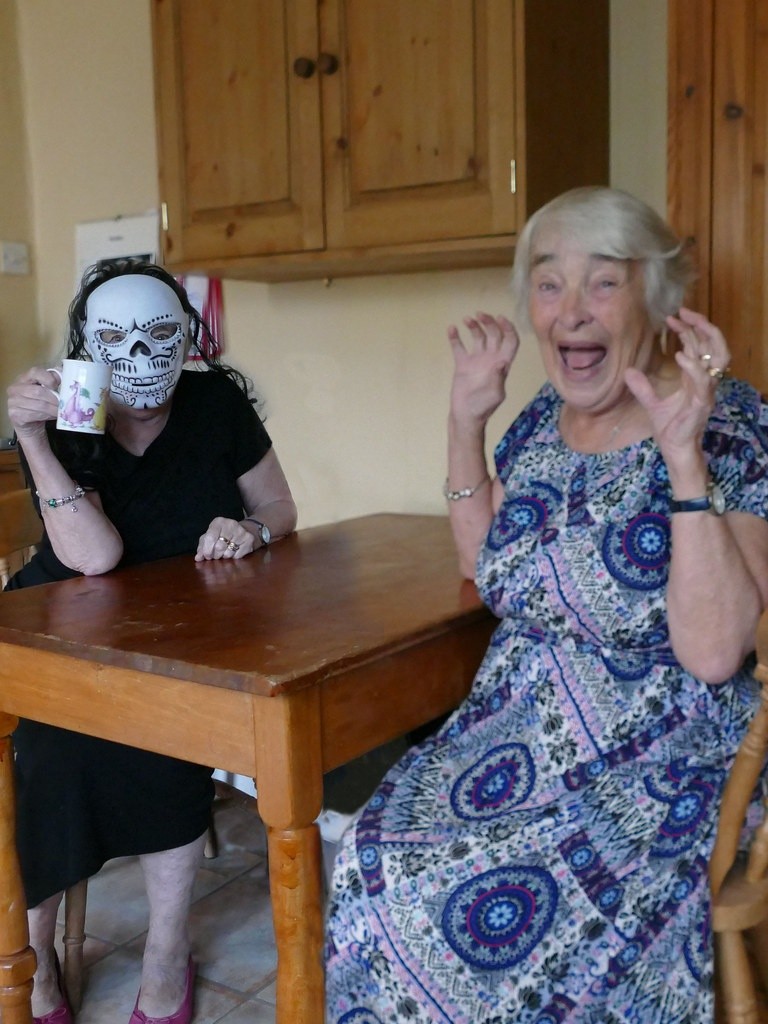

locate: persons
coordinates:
[312,189,768,1024]
[3,255,298,1023]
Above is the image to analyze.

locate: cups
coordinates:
[42,358,113,435]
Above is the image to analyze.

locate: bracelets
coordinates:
[35,479,85,513]
[441,473,491,503]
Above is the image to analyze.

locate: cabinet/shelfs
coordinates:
[152,0,607,284]
[667,0,768,397]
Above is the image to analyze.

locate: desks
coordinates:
[0,512,512,1024]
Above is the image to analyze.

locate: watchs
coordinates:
[245,518,271,546]
[668,482,726,517]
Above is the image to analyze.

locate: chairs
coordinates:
[0,493,218,1006]
[705,612,768,1024]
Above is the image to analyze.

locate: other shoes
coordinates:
[129,953,194,1024]
[32,947,72,1024]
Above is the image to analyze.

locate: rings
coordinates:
[219,537,231,545]
[698,354,712,360]
[228,542,239,552]
[706,367,724,379]
